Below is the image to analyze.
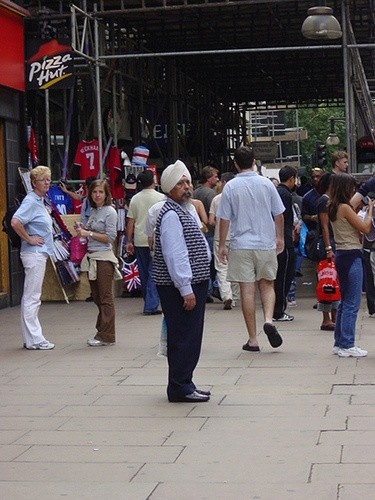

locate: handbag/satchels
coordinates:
[304,230,335,262]
[316,257,341,304]
[69,234,89,264]
[298,222,309,257]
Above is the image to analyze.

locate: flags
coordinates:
[124,260,142,292]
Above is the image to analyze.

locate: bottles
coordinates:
[77,221,87,244]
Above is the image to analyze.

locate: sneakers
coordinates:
[87,337,115,346]
[338,345,369,357]
[24,339,55,350]
[332,345,339,355]
[272,312,294,321]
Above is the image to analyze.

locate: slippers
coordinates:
[263,322,283,348]
[243,339,259,351]
[320,323,336,330]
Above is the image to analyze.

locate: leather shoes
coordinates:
[169,390,210,402]
[195,389,210,394]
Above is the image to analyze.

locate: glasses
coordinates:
[35,177,51,182]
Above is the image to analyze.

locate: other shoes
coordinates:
[223,298,232,309]
[143,309,162,315]
[287,300,296,306]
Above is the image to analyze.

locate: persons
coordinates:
[210,171,240,309]
[189,198,208,224]
[151,160,211,403]
[77,178,123,345]
[60,176,96,301]
[144,200,203,258]
[215,147,286,351]
[11,166,54,349]
[270,166,341,330]
[191,166,223,304]
[126,170,162,316]
[326,172,375,357]
[330,151,375,317]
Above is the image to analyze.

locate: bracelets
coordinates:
[89,232,93,237]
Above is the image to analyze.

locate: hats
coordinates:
[124,174,137,189]
[138,170,154,185]
